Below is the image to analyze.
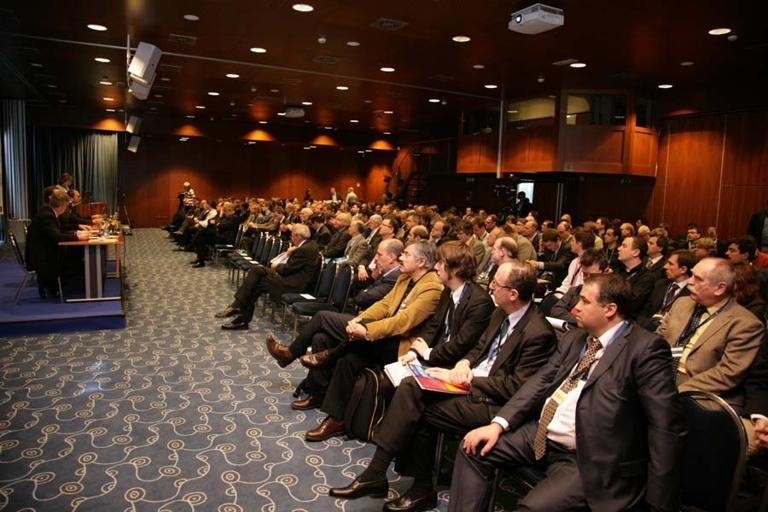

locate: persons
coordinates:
[447,272,679,511]
[162,179,768,463]
[24,171,109,298]
[324,261,556,512]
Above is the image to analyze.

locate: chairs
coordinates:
[431,424,547,512]
[213,223,291,288]
[23,221,28,236]
[8,231,66,306]
[263,255,354,340]
[679,388,768,512]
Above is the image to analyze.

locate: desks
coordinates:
[59,221,123,301]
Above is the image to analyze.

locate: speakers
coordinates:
[126,115,143,134]
[129,41,163,100]
[125,135,141,153]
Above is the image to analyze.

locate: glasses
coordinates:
[492,278,511,290]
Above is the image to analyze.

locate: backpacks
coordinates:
[342,366,388,444]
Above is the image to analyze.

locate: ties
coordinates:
[569,257,582,287]
[485,316,513,367]
[645,259,655,271]
[438,295,458,344]
[670,301,708,382]
[661,283,681,309]
[551,252,556,261]
[528,337,605,462]
[367,231,374,243]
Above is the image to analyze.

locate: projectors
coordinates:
[507,3,564,34]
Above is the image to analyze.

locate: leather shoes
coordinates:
[298,349,337,370]
[190,259,206,268]
[222,317,249,330]
[328,475,389,499]
[265,330,296,369]
[161,225,188,251]
[214,305,241,318]
[304,414,345,441]
[290,394,322,410]
[382,487,438,511]
[37,287,65,299]
